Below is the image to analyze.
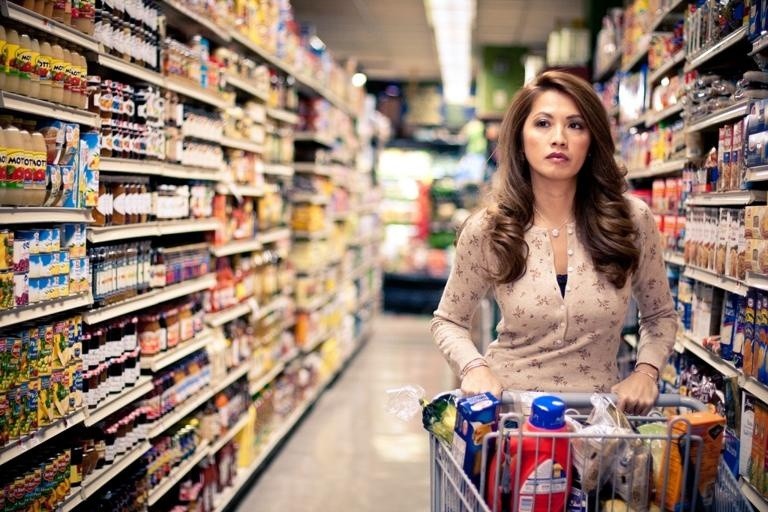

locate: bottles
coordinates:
[162,307,181,347]
[86,240,154,309]
[80,315,141,408]
[192,300,203,333]
[0,18,89,108]
[88,75,148,124]
[154,242,210,288]
[6,0,96,35]
[139,314,162,357]
[69,405,148,486]
[98,118,147,159]
[147,349,213,420]
[97,0,163,71]
[0,114,46,207]
[179,303,195,339]
[158,313,168,352]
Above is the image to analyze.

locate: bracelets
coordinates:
[460,362,489,375]
[631,369,659,384]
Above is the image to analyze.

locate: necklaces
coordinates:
[533,202,575,237]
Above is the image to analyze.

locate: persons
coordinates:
[429,69,679,417]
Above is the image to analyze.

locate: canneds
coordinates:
[0,451,72,512]
[0,315,83,450]
[91,182,177,228]
[0,224,89,310]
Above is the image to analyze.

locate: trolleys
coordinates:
[429,391,754,512]
